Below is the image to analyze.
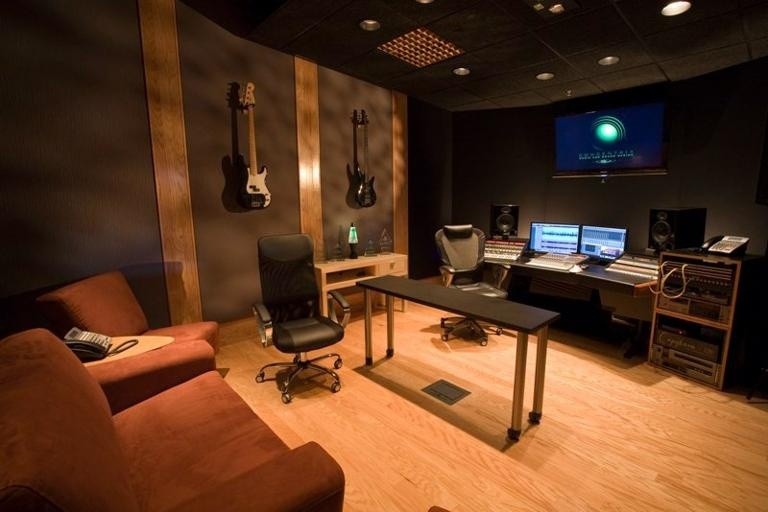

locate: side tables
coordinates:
[82,335,175,368]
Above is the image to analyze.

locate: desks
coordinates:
[510,253,660,359]
[356,275,561,442]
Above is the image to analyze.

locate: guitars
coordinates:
[355,108,377,206]
[237,82,271,209]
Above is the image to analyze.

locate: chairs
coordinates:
[435,224,511,346]
[252,233,351,404]
[36,272,220,358]
[427,506,450,512]
[0,327,345,512]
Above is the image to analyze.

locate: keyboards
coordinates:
[525,258,575,271]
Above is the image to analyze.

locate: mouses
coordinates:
[581,264,590,270]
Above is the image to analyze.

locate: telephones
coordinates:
[701,236,751,255]
[63,327,111,361]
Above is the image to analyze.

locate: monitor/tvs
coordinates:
[579,224,629,266]
[552,96,672,176]
[529,222,580,254]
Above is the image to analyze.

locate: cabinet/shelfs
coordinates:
[312,251,408,327]
[647,248,767,391]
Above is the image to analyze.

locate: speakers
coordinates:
[490,205,520,239]
[648,208,706,256]
